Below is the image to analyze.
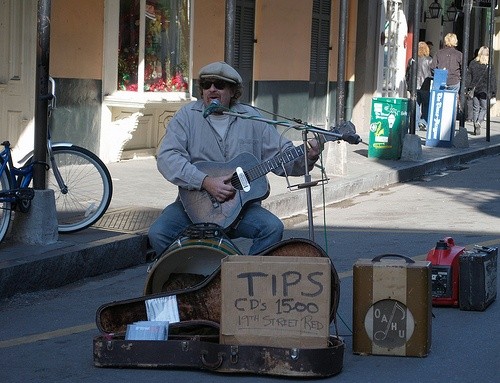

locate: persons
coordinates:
[431,33,463,104]
[465,46,497,134]
[149,62,320,260]
[406,42,433,131]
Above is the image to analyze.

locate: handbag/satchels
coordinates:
[466,88,475,101]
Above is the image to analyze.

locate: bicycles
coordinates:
[0,75,113,245]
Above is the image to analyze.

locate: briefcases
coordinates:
[351,252,433,358]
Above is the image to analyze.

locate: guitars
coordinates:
[178,118,356,231]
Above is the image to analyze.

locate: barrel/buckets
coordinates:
[424,236,465,306]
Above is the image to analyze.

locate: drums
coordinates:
[144,235,244,299]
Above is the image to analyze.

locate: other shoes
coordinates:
[418,123,426,131]
[473,122,482,135]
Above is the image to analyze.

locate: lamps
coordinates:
[441,2,459,26]
[424,0,443,23]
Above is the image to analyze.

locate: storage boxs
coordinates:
[458,244,499,312]
[221,255,331,349]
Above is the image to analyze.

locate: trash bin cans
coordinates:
[425,69,459,148]
[368,97,411,160]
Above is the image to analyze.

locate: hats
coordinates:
[199,61,243,85]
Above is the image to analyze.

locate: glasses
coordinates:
[200,79,226,90]
